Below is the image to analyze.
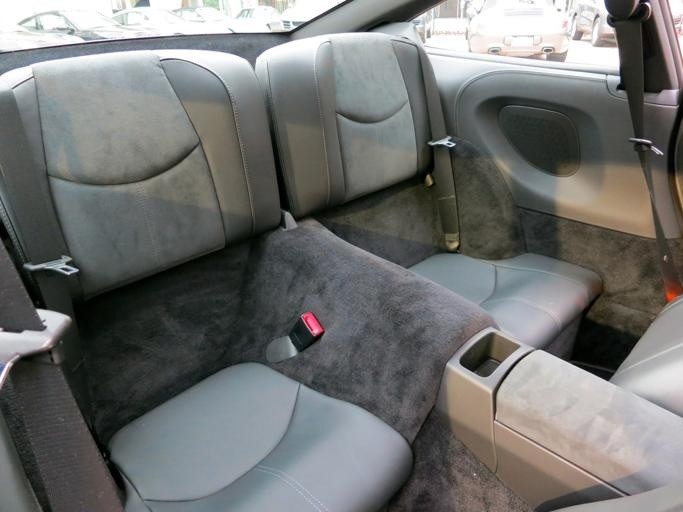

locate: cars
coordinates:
[409,7,435,40]
[0,0,343,51]
[461,0,681,61]
